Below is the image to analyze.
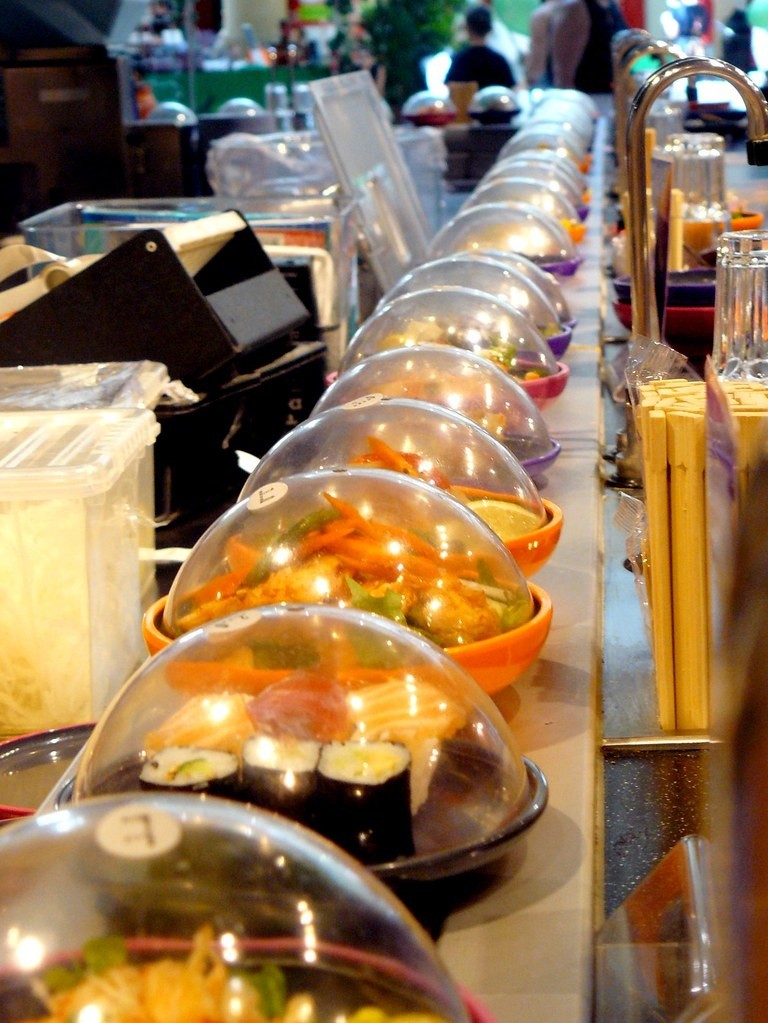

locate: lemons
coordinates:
[460,499,542,543]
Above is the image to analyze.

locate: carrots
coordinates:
[191,492,494,597]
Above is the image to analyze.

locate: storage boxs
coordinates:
[19,200,361,334]
[1,359,173,741]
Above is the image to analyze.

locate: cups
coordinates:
[662,132,733,224]
[710,229,768,383]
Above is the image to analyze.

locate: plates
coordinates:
[2,109,600,1022]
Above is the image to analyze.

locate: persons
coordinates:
[280,21,316,58]
[443,6,515,90]
[722,10,756,74]
[525,0,630,94]
[134,0,174,43]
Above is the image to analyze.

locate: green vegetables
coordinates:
[27,931,288,1021]
[340,577,445,668]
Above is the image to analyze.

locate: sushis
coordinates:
[135,732,420,870]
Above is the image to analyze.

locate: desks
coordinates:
[135,68,340,112]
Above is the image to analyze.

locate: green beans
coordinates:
[239,507,342,586]
